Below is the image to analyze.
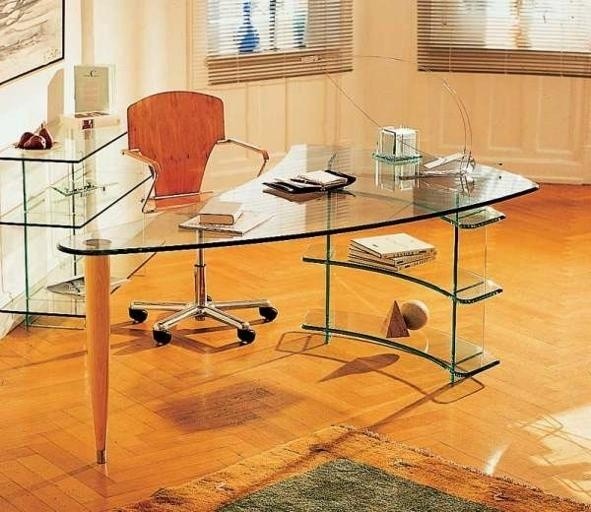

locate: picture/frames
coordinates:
[0,1,66,85]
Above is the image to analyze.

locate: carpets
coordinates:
[105,424,591,511]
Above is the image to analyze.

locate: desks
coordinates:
[57,145,540,468]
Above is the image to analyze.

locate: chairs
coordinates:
[121,89,278,343]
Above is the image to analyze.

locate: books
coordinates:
[263,168,357,196]
[59,62,121,131]
[347,233,439,273]
[197,198,245,225]
[179,209,274,237]
[47,273,132,297]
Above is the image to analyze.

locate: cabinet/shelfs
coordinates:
[1,119,167,331]
